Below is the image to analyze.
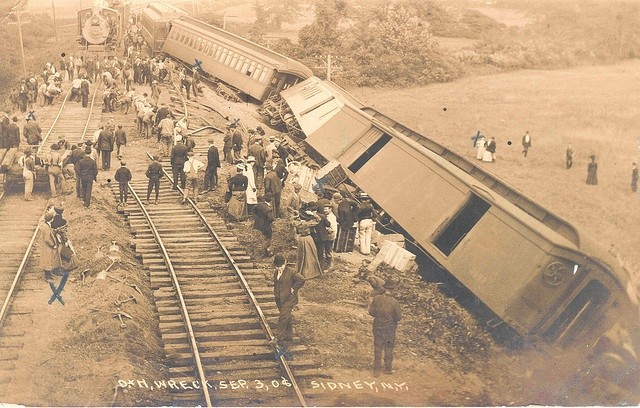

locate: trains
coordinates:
[135,1,633,374]
[74,1,131,59]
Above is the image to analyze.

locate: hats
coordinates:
[50,143,58,150]
[29,77,36,82]
[187,152,193,156]
[247,156,258,161]
[11,116,18,121]
[24,148,33,155]
[85,146,92,152]
[332,193,342,200]
[255,135,262,140]
[247,130,255,133]
[294,184,302,189]
[272,149,278,152]
[236,163,245,169]
[359,193,369,198]
[268,136,277,141]
[175,135,183,142]
[384,279,395,289]
[77,141,83,144]
[44,213,53,220]
[229,123,238,127]
[263,162,274,167]
[273,154,280,158]
[224,122,231,127]
[85,139,93,145]
[273,255,285,267]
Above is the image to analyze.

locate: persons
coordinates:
[266,135,279,156]
[123,19,144,54]
[202,139,220,193]
[332,192,359,230]
[0,117,10,149]
[46,205,55,211]
[225,163,249,223]
[22,117,43,145]
[265,149,279,164]
[113,160,132,207]
[262,160,282,218]
[170,135,187,190]
[250,126,265,137]
[272,255,306,341]
[475,135,486,160]
[143,155,163,205]
[51,207,77,269]
[482,137,496,162]
[521,131,531,158]
[484,138,496,160]
[367,281,401,378]
[287,183,337,280]
[21,147,36,201]
[565,143,574,168]
[173,127,183,145]
[357,192,378,256]
[132,54,200,100]
[270,154,289,183]
[181,151,204,204]
[9,54,133,115]
[247,136,266,166]
[43,116,115,207]
[251,192,275,257]
[630,162,639,192]
[115,124,127,160]
[229,123,243,160]
[223,123,229,161]
[41,210,60,280]
[274,141,289,166]
[7,116,20,150]
[584,154,598,185]
[242,155,258,212]
[222,123,233,166]
[133,100,173,157]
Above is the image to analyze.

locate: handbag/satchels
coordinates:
[225,191,233,203]
